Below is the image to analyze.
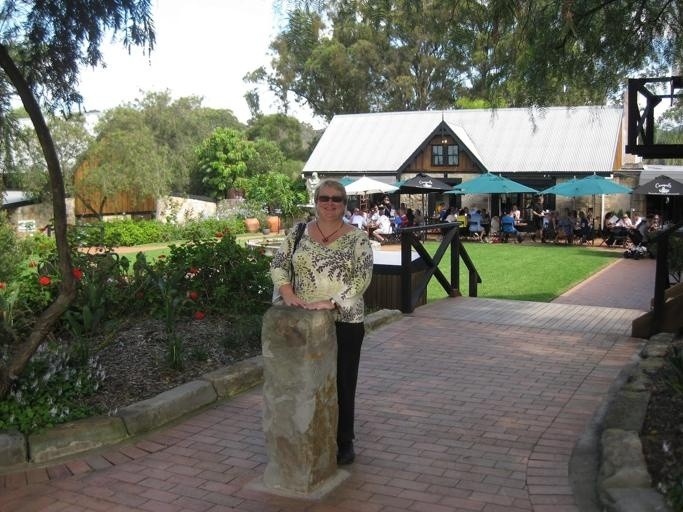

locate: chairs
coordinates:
[349,205,667,249]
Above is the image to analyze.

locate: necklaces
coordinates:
[314,219,343,243]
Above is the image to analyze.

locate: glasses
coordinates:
[318,196,343,202]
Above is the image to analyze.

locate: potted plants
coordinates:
[231,167,307,236]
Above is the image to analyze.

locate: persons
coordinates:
[271,180,373,464]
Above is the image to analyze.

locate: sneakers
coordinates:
[337,443,356,465]
